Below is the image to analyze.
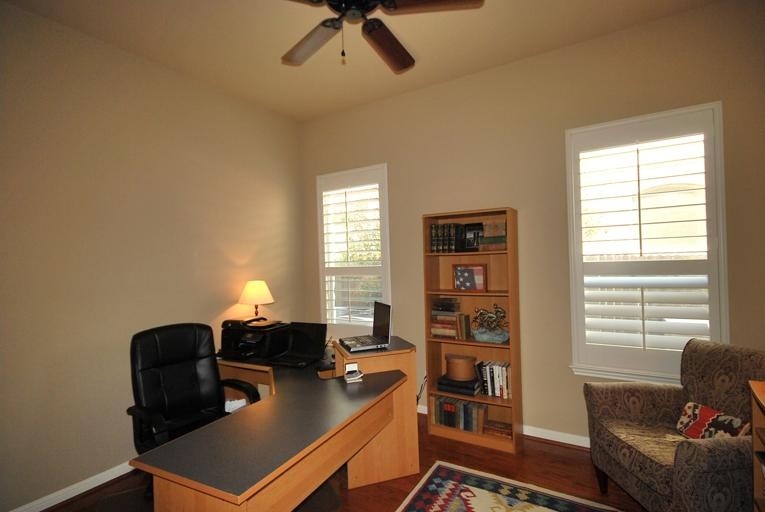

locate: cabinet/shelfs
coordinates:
[748,378,765,512]
[421,207,525,456]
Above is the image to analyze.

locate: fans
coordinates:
[280,0,486,75]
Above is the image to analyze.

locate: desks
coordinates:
[130,334,420,511]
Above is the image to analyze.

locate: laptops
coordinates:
[339,301,392,353]
[258,322,327,368]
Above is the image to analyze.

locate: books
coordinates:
[435,353,511,444]
[428,295,472,342]
[425,224,510,255]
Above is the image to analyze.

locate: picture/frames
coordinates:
[452,263,488,293]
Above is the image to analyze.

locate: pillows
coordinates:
[671,401,752,446]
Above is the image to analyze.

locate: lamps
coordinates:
[237,279,276,318]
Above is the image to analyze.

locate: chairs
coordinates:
[582,337,765,512]
[125,323,262,504]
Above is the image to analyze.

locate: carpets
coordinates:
[394,459,625,512]
